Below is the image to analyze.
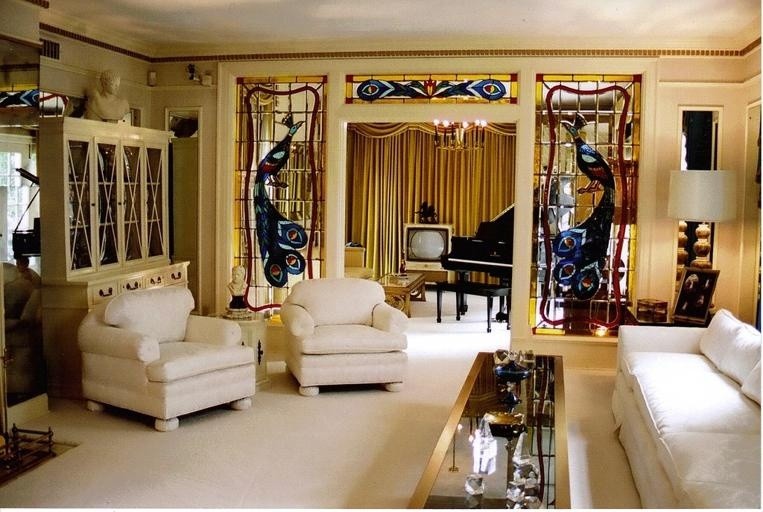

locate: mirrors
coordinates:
[670,104,724,308]
[164,106,203,316]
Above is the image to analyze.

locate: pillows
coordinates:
[288,278,385,327]
[699,308,743,368]
[104,285,195,344]
[741,360,761,406]
[718,325,761,386]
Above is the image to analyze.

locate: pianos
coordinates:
[441,172,576,323]
[12,168,41,257]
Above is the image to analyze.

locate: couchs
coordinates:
[304,247,374,281]
[611,308,761,508]
[3,262,41,400]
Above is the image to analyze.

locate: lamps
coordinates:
[667,170,737,315]
[433,118,487,152]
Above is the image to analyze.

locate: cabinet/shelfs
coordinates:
[37,116,190,401]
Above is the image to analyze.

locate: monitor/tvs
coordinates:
[403,223,452,270]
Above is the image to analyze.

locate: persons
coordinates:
[227,266,248,296]
[86,69,130,122]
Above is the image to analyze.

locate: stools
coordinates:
[436,281,511,333]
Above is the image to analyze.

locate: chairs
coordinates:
[77,285,256,431]
[280,277,409,397]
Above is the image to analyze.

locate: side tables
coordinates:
[624,306,713,328]
[207,312,270,386]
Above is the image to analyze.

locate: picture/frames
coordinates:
[670,267,720,325]
